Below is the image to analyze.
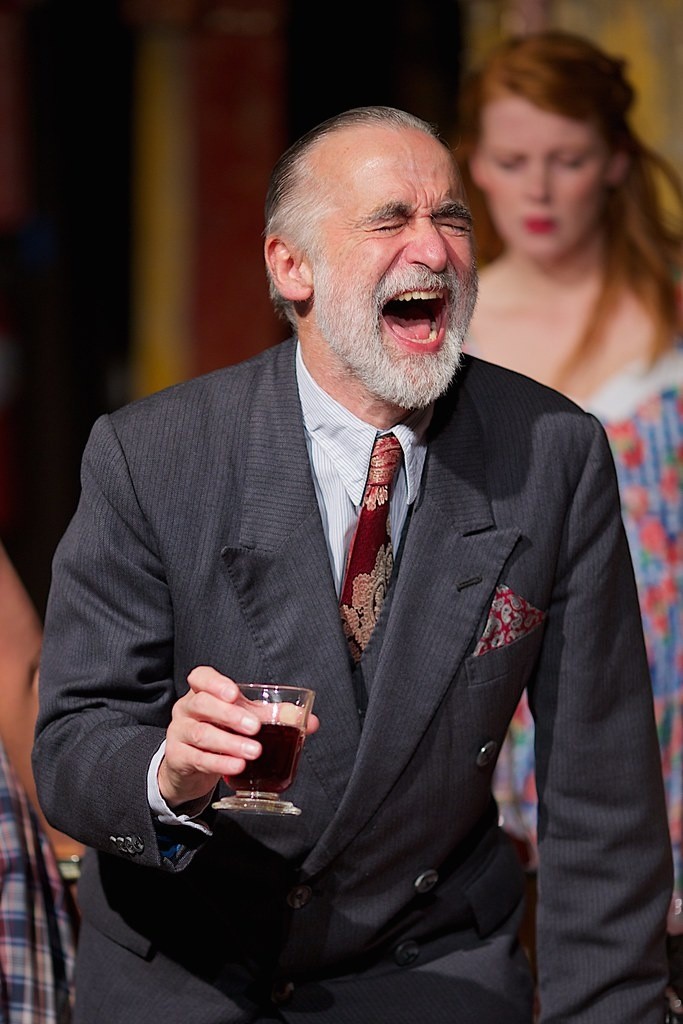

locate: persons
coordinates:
[0,544,84,1024]
[31,105,674,1024]
[454,28,683,1024]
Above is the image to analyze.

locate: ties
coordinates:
[337,432,404,671]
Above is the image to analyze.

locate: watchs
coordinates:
[53,854,82,883]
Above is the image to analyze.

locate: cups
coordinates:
[212,684,315,816]
